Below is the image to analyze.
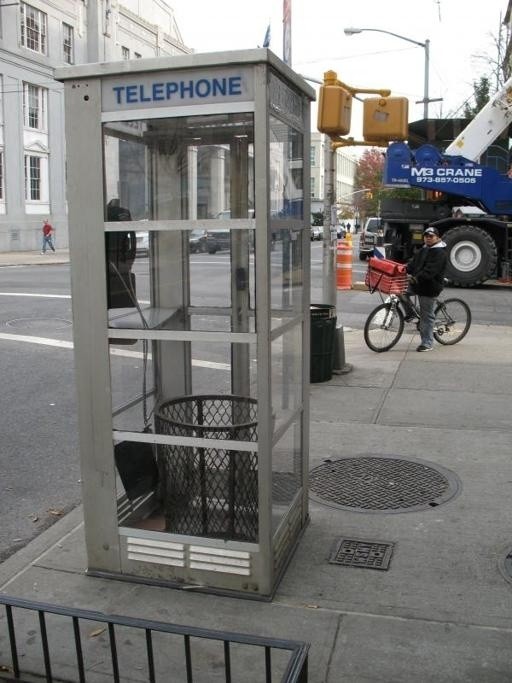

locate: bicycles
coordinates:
[363,257,472,353]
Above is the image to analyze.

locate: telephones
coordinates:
[108,207,137,274]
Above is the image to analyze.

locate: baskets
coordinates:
[364,270,411,296]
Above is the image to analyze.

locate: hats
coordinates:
[422,227,439,236]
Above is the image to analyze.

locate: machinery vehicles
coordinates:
[375,68,512,289]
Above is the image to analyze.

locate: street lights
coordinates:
[343,27,430,120]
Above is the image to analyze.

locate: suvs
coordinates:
[206,207,275,254]
[358,218,385,260]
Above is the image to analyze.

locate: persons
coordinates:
[341,222,345,228]
[40,218,55,255]
[397,228,449,353]
[347,222,351,233]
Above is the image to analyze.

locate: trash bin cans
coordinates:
[308,303,337,383]
[158,394,276,544]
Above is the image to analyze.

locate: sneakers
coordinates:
[417,344,433,352]
[404,314,415,322]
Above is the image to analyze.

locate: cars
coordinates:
[128,219,150,258]
[290,217,345,241]
[188,227,208,254]
